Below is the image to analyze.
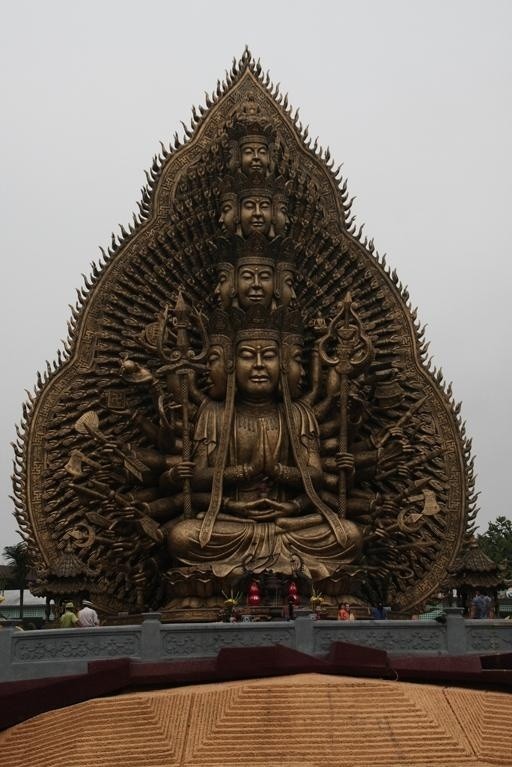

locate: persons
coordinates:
[280,596,300,622]
[58,601,80,628]
[473,589,486,617]
[88,116,414,577]
[337,602,355,619]
[368,601,389,619]
[480,592,491,617]
[339,603,349,619]
[77,599,99,626]
